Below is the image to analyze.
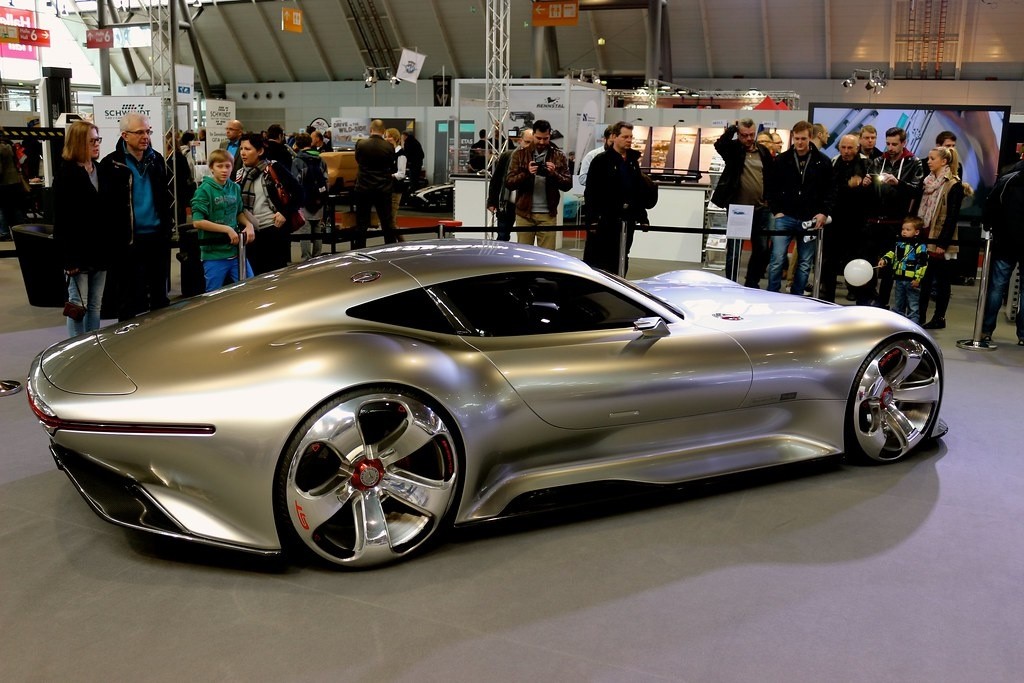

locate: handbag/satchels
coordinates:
[892,260,899,270]
[641,173,658,208]
[62,274,87,321]
[267,163,305,233]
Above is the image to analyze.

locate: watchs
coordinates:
[20,140,46,183]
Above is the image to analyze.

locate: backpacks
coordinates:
[297,155,329,202]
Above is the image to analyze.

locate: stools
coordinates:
[439,220,463,239]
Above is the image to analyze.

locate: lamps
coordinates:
[564,69,600,84]
[674,88,700,97]
[361,68,379,89]
[863,67,888,94]
[385,70,401,87]
[841,70,858,88]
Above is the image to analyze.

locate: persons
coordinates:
[258,122,294,172]
[816,123,975,330]
[766,120,833,295]
[507,118,572,250]
[48,118,115,339]
[401,131,426,188]
[101,113,173,326]
[756,130,790,280]
[353,119,397,247]
[982,145,1024,346]
[466,128,515,173]
[293,131,330,262]
[579,120,650,278]
[711,117,774,290]
[0,141,39,241]
[163,125,207,184]
[567,150,575,175]
[305,126,335,154]
[190,149,256,290]
[219,119,247,182]
[488,129,533,241]
[232,132,302,277]
[786,123,831,292]
[383,127,407,241]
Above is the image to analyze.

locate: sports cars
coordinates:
[24,238,949,576]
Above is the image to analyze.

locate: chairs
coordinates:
[13,223,68,309]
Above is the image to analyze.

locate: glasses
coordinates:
[89,137,102,145]
[125,130,154,136]
[225,127,239,131]
[757,141,771,145]
[522,138,531,144]
[737,131,755,139]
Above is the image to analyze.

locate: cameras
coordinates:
[533,162,550,177]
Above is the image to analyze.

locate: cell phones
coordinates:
[869,174,884,183]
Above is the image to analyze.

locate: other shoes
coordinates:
[929,287,937,302]
[846,290,857,301]
[923,311,945,329]
[917,310,926,325]
[786,279,813,295]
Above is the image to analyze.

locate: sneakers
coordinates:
[1018,340,1024,347]
